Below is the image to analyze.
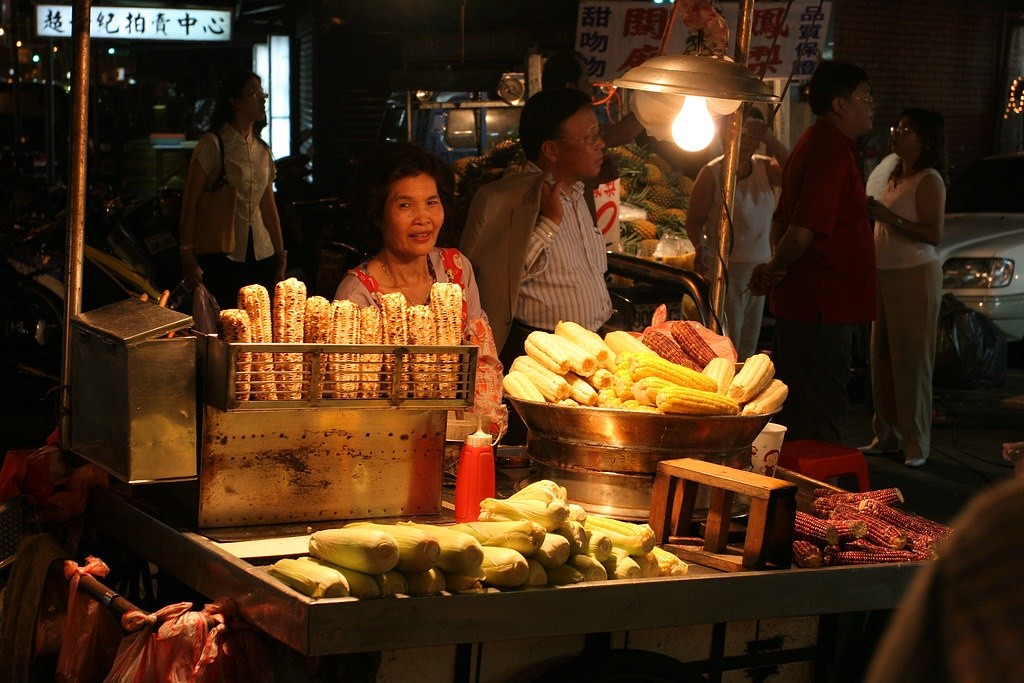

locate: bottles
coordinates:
[455,404,507,522]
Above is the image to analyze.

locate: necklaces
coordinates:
[380,256,433,307]
[738,160,754,181]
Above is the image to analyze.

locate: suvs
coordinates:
[373,89,526,216]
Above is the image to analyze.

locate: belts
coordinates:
[511,324,532,338]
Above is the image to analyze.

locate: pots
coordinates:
[498,390,784,523]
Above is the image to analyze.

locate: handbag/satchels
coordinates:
[186,132,239,255]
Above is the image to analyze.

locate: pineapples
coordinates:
[595,143,700,269]
[446,136,526,203]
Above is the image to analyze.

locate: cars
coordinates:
[938,151,1024,344]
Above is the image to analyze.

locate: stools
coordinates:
[782,439,870,497]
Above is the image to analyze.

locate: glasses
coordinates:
[234,91,269,99]
[838,94,876,107]
[890,126,917,138]
[555,128,607,147]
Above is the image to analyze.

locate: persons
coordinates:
[177,64,285,319]
[457,87,618,451]
[686,101,790,361]
[857,106,947,468]
[331,160,508,448]
[746,57,880,451]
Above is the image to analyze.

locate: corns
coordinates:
[502,320,788,416]
[789,486,957,567]
[215,277,464,401]
[268,480,690,598]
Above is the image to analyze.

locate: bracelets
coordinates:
[180,244,193,252]
[695,243,710,250]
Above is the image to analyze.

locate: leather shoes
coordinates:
[905,456,926,466]
[857,443,901,454]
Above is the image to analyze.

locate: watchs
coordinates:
[894,217,903,228]
